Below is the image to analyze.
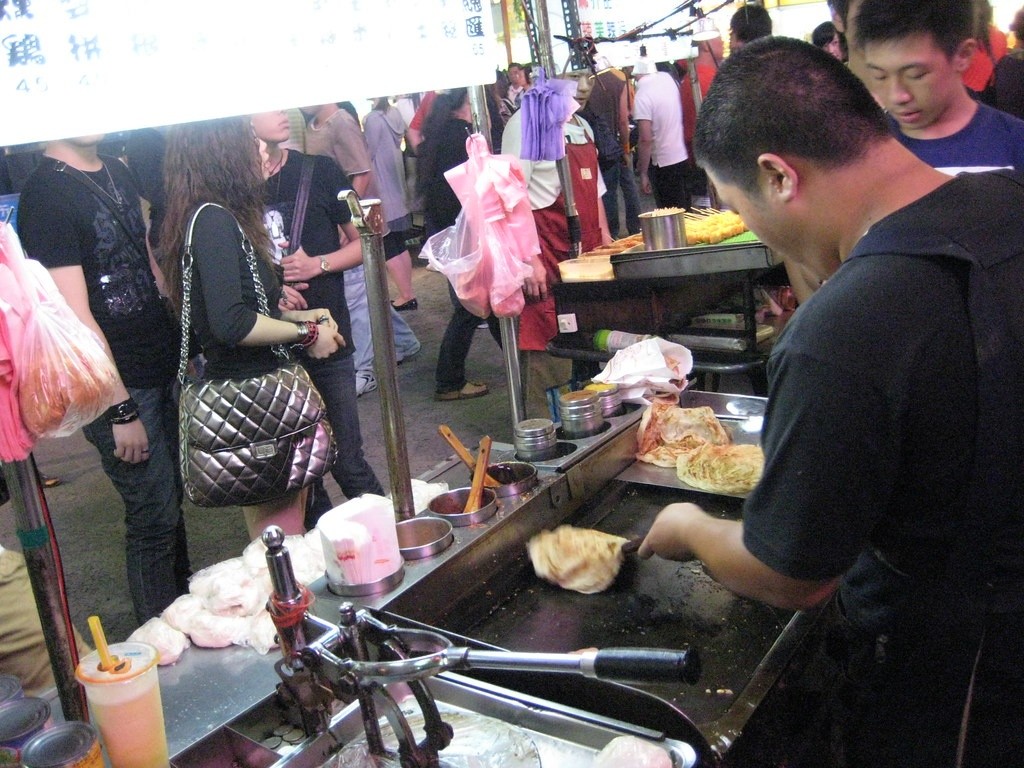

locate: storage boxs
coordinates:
[580,249,631,259]
[558,257,612,283]
[593,242,640,249]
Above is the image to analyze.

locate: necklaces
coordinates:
[42,152,122,205]
[262,149,284,237]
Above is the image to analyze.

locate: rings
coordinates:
[316,314,330,324]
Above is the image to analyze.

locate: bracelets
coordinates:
[294,322,309,341]
[106,397,140,424]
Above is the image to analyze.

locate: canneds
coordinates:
[0,674,105,768]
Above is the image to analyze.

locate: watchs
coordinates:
[290,320,319,353]
[317,256,330,275]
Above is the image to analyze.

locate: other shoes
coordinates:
[391,298,417,312]
[433,382,490,400]
[39,472,63,489]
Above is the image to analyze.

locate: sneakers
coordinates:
[356,371,378,396]
[395,340,423,364]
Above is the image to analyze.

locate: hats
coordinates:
[631,63,657,74]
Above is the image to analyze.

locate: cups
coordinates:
[324,555,405,597]
[468,460,538,498]
[427,486,498,527]
[395,516,453,560]
[637,209,688,251]
[74,640,171,768]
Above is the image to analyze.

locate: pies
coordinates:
[526,524,631,594]
[637,403,764,493]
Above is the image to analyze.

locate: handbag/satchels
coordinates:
[180,203,338,506]
[0,222,130,436]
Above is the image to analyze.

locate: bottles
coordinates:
[583,383,623,418]
[557,390,604,439]
[511,418,557,462]
[591,330,653,354]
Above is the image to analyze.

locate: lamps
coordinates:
[664,28,692,59]
[633,40,659,77]
[689,7,722,43]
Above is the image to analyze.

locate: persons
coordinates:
[0,464,95,695]
[638,0,1024,768]
[287,64,636,398]
[16,133,193,629]
[153,119,343,542]
[242,113,383,533]
